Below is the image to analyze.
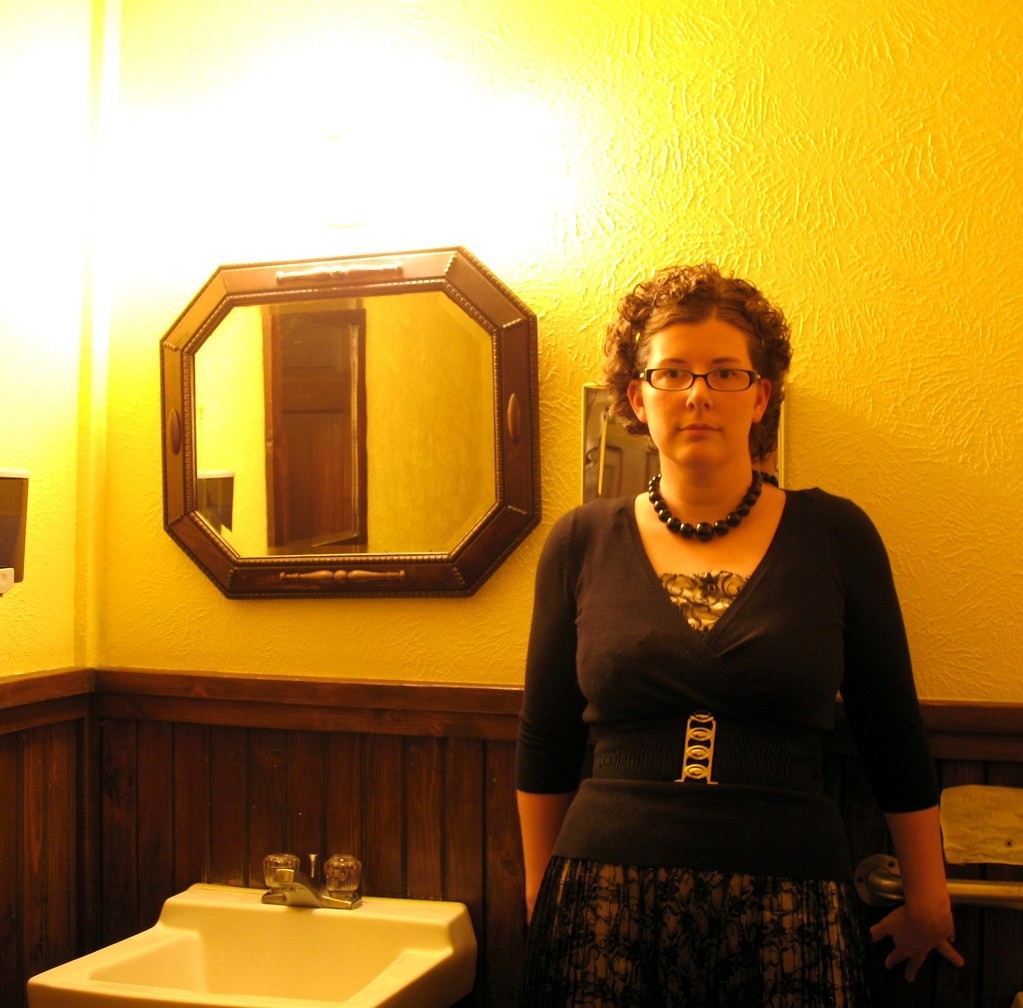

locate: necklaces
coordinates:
[762,471,778,487]
[648,470,762,542]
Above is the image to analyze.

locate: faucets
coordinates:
[274,868,322,907]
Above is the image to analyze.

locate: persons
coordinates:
[514,261,963,1008]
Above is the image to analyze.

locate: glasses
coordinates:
[633,368,762,392]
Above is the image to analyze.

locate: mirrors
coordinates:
[158,245,541,600]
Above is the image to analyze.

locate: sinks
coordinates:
[27,883,477,1008]
